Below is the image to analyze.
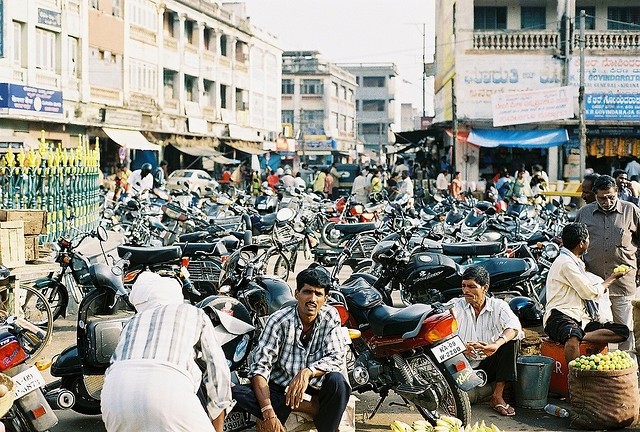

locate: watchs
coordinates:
[499,334,508,344]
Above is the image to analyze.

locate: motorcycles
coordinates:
[254,177,411,226]
[49,263,255,414]
[0,275,76,431]
[99,189,255,238]
[412,187,578,233]
[307,235,488,428]
[24,198,189,327]
[218,238,369,391]
[355,225,541,309]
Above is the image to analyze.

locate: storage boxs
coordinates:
[0,209,45,235]
[25,236,39,263]
[0,220,25,269]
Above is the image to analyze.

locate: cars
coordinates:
[165,169,221,198]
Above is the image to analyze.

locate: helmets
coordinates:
[284,169,291,175]
[141,163,152,170]
[509,297,545,328]
[277,168,284,175]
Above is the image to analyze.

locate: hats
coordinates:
[129,271,184,314]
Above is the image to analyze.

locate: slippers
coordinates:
[492,403,515,415]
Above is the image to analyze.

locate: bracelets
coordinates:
[261,404,273,413]
[262,415,277,423]
[307,365,316,378]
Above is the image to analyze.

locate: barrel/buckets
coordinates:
[514,355,553,408]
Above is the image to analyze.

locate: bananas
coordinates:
[389,415,500,432]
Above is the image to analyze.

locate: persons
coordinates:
[301,163,315,188]
[408,154,455,178]
[153,161,170,189]
[330,162,345,201]
[484,163,553,205]
[630,175,640,198]
[361,158,408,173]
[250,164,294,197]
[581,173,600,205]
[388,172,401,194]
[397,170,414,197]
[294,172,306,193]
[446,266,523,416]
[449,171,465,200]
[436,170,449,198]
[351,171,369,197]
[323,169,334,195]
[542,222,634,368]
[313,169,326,192]
[98,164,130,201]
[125,163,154,199]
[370,170,382,193]
[218,167,233,199]
[100,271,238,431]
[573,175,639,353]
[624,156,640,181]
[310,166,318,184]
[612,170,640,206]
[231,268,352,432]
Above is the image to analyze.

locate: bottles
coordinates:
[545,404,569,419]
